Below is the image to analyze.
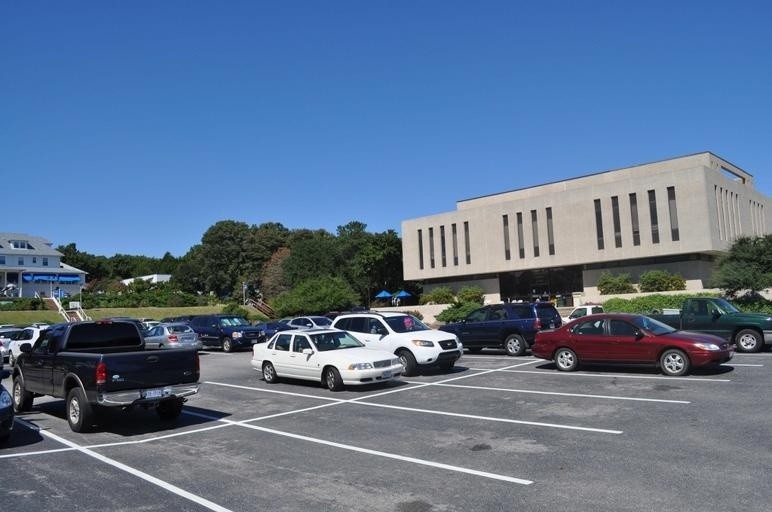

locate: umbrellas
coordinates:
[375,289,393,298]
[397,290,412,297]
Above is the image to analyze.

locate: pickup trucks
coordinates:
[646,297,772,353]
[11,319,201,433]
[560,304,606,329]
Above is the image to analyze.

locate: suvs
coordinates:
[173,313,267,352]
[328,311,463,377]
[0,322,50,371]
[438,302,563,357]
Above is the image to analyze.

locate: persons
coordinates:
[392,297,401,307]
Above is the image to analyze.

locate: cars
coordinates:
[143,323,203,352]
[254,315,334,342]
[250,329,407,392]
[126,317,178,336]
[531,312,734,376]
[0,369,14,441]
